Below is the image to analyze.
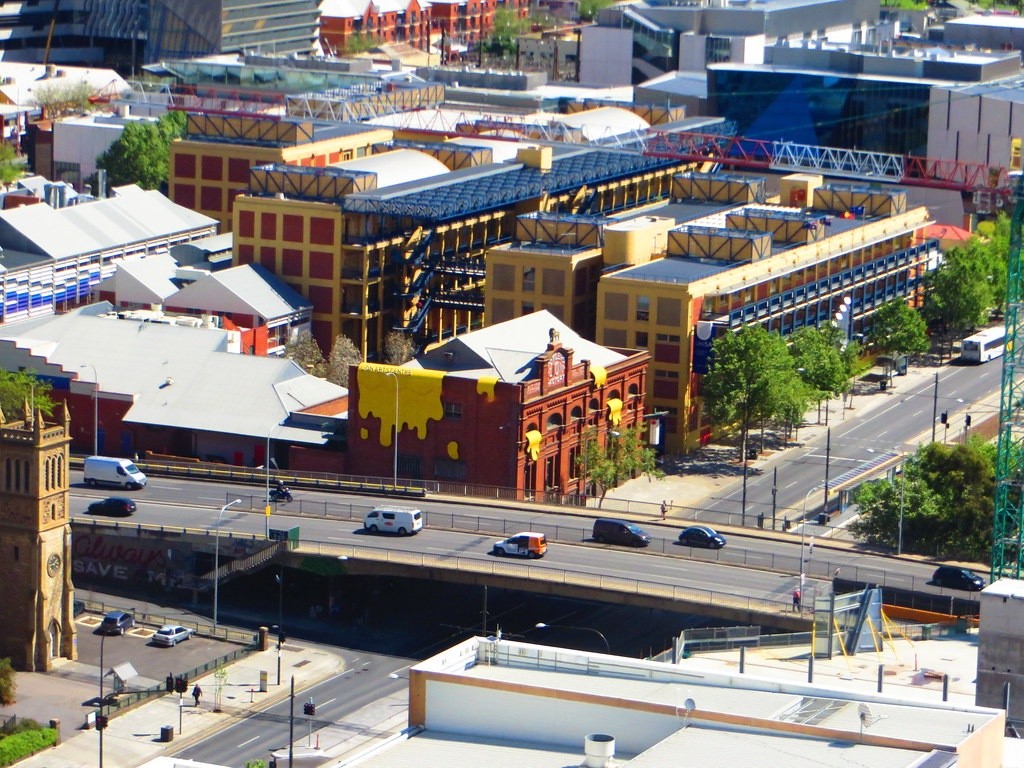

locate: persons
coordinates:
[661,500,668,519]
[277,480,287,498]
[792,586,801,613]
[193,684,202,707]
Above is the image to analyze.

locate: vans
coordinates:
[83,456,147,490]
[592,518,651,547]
[364,506,422,536]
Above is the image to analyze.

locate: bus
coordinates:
[961,327,1014,363]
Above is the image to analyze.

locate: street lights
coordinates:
[81,363,97,455]
[265,420,285,540]
[213,497,241,636]
[535,623,610,655]
[799,487,818,612]
[893,445,904,555]
[385,372,398,491]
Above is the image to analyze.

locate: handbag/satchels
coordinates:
[192,692,199,696]
[278,491,282,496]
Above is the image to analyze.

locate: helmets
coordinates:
[279,480,283,484]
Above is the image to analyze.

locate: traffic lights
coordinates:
[166,677,173,692]
[96,716,108,731]
[176,678,187,693]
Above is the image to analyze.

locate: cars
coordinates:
[933,566,985,592]
[100,611,135,635]
[679,525,727,549]
[88,496,137,515]
[151,624,193,647]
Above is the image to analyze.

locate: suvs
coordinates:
[493,531,548,559]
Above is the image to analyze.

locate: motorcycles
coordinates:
[269,487,293,502]
